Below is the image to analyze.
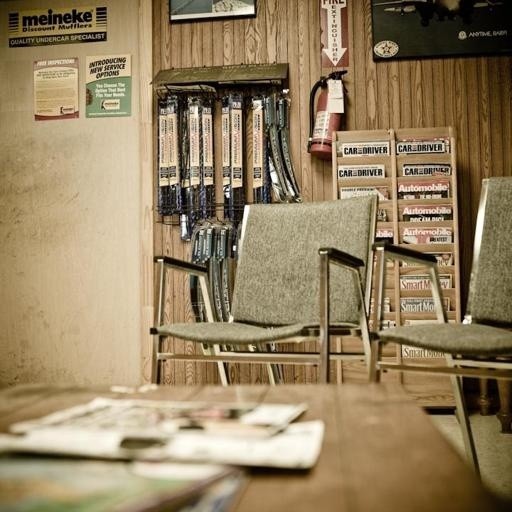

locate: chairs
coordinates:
[372,177,512,484]
[148,199,379,393]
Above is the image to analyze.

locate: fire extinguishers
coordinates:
[308,70,347,162]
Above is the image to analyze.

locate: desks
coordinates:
[0,382,502,511]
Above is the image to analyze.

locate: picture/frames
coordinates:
[168,1,256,22]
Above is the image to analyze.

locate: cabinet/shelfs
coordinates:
[332,126,463,407]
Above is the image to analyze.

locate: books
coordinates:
[337,136,455,358]
